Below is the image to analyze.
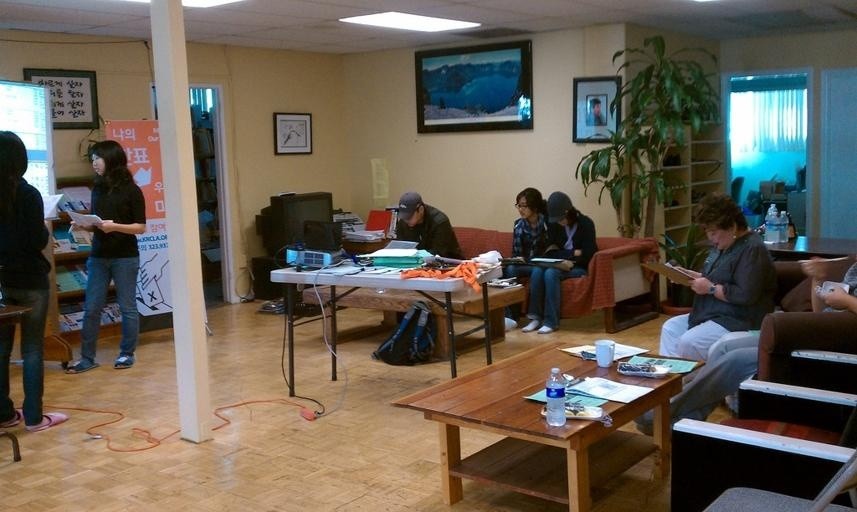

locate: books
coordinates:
[43,185,123,334]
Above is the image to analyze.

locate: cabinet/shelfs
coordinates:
[44,175,124,361]
[631,119,730,304]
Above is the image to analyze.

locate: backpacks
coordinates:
[372,297,437,366]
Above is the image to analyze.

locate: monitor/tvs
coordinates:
[261,190,333,259]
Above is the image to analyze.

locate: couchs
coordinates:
[758,259,857,381]
[451,228,661,333]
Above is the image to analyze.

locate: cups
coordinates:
[596,339,616,370]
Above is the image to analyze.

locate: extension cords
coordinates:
[299,407,315,420]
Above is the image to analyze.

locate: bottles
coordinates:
[763,202,791,246]
[545,368,567,427]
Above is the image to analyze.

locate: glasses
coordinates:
[514,203,529,210]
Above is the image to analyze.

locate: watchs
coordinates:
[708,282,719,295]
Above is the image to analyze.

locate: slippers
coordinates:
[27,411,69,433]
[114,354,134,369]
[67,358,100,373]
[3,408,23,427]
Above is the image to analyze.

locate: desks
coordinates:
[342,239,390,256]
[269,258,504,398]
[765,234,857,262]
[0,303,35,461]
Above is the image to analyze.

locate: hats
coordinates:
[399,192,421,220]
[547,191,572,223]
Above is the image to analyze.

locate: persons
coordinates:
[64,140,147,374]
[633,256,856,434]
[0,130,70,432]
[522,190,598,334]
[500,187,553,331]
[380,191,462,325]
[658,191,779,364]
[586,98,606,125]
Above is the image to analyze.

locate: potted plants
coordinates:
[575,34,722,316]
[660,221,715,317]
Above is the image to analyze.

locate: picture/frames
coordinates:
[571,75,622,143]
[273,112,312,155]
[413,36,534,134]
[23,66,101,130]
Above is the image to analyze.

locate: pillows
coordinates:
[799,256,854,315]
[781,277,812,313]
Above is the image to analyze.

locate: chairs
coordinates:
[671,350,857,512]
